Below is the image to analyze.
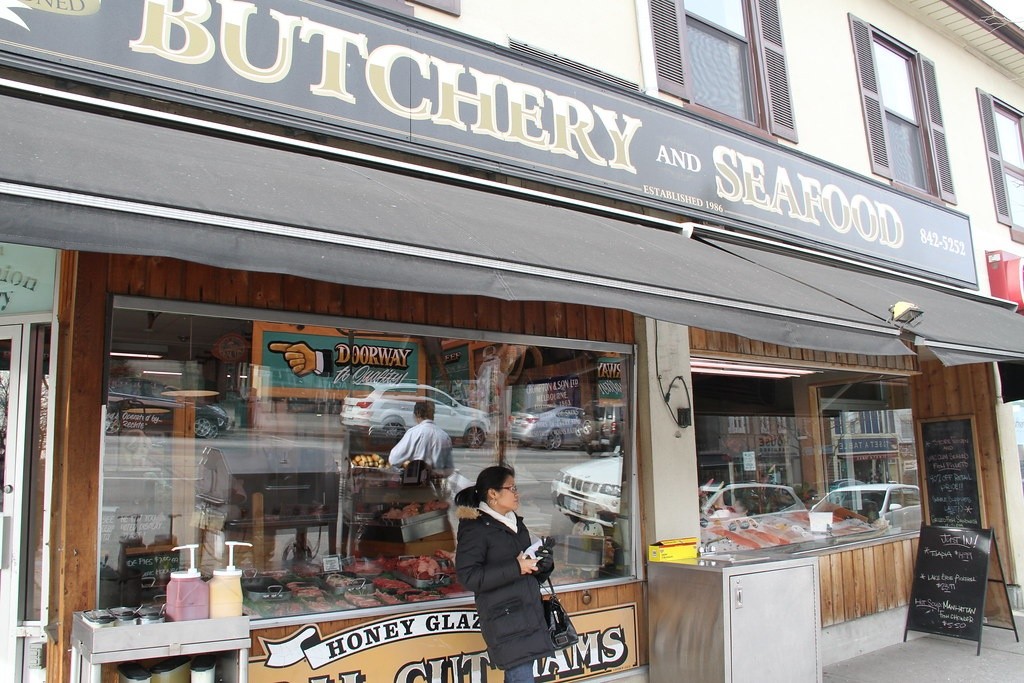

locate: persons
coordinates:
[115,428,166,517]
[716,433,747,509]
[312,450,339,510]
[238,473,290,516]
[550,489,581,579]
[454,458,555,682]
[389,400,454,498]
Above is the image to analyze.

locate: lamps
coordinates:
[160,314,221,396]
[889,301,923,324]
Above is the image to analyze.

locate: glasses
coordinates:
[485,484,518,493]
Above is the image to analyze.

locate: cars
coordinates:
[699,478,808,524]
[549,446,624,537]
[581,399,626,457]
[103,372,231,439]
[511,405,585,451]
[807,476,922,537]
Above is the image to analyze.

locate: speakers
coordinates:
[678,407,692,426]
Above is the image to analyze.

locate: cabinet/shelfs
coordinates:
[70,609,251,683]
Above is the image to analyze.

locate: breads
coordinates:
[352,454,390,468]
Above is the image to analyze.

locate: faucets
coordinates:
[705,536,734,552]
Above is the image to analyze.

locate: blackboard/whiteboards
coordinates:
[906,524,1016,642]
[917,414,988,529]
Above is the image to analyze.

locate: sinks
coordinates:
[697,554,764,562]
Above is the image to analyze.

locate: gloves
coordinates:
[534,535,556,583]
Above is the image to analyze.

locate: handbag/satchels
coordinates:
[537,578,579,650]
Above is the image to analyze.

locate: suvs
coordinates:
[339,380,492,450]
